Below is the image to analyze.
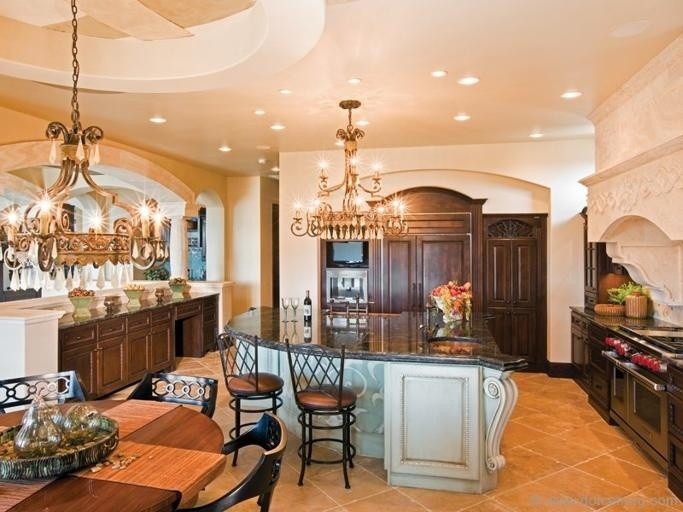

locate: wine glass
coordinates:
[281,298,291,323]
[291,298,299,323]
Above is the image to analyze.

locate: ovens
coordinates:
[602,348,668,471]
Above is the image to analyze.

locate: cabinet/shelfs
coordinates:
[583,223,629,309]
[126,305,172,385]
[182,295,219,358]
[570,311,609,426]
[666,365,682,502]
[57,316,126,403]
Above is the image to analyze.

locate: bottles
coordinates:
[304,320,312,343]
[303,290,312,321]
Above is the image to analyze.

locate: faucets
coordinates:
[356,313,362,341]
[354,293,360,313]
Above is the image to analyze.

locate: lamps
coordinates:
[290,100,409,239]
[0,0,170,272]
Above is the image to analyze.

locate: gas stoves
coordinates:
[645,335,683,353]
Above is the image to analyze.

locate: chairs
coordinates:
[214,332,283,467]
[126,372,218,420]
[0,371,85,416]
[173,412,287,512]
[285,338,356,490]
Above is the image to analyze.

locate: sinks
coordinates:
[328,311,400,318]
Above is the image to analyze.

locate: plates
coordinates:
[1,417,120,481]
